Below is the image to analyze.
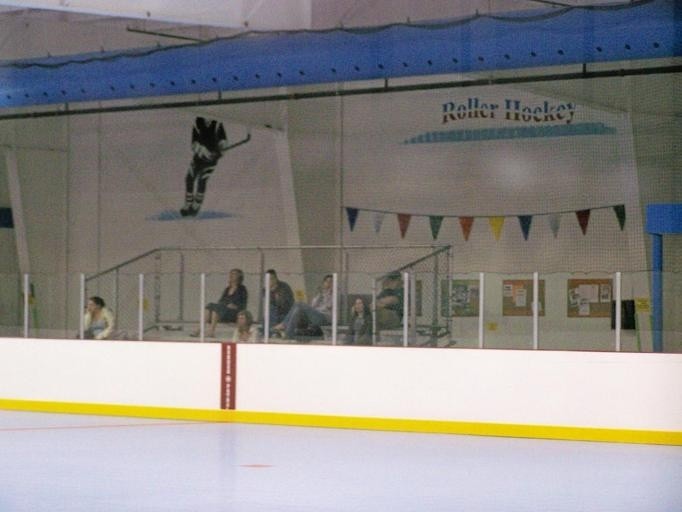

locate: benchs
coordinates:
[141,314,409,346]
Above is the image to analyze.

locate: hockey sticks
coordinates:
[210,126,251,152]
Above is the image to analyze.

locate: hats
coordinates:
[388,271,400,277]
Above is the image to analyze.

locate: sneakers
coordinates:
[190,201,201,215]
[181,201,192,214]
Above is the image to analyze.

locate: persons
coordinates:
[343,298,378,346]
[234,310,258,347]
[78,295,115,340]
[370,270,405,327]
[179,115,229,218]
[273,275,346,340]
[191,270,247,337]
[260,270,294,339]
